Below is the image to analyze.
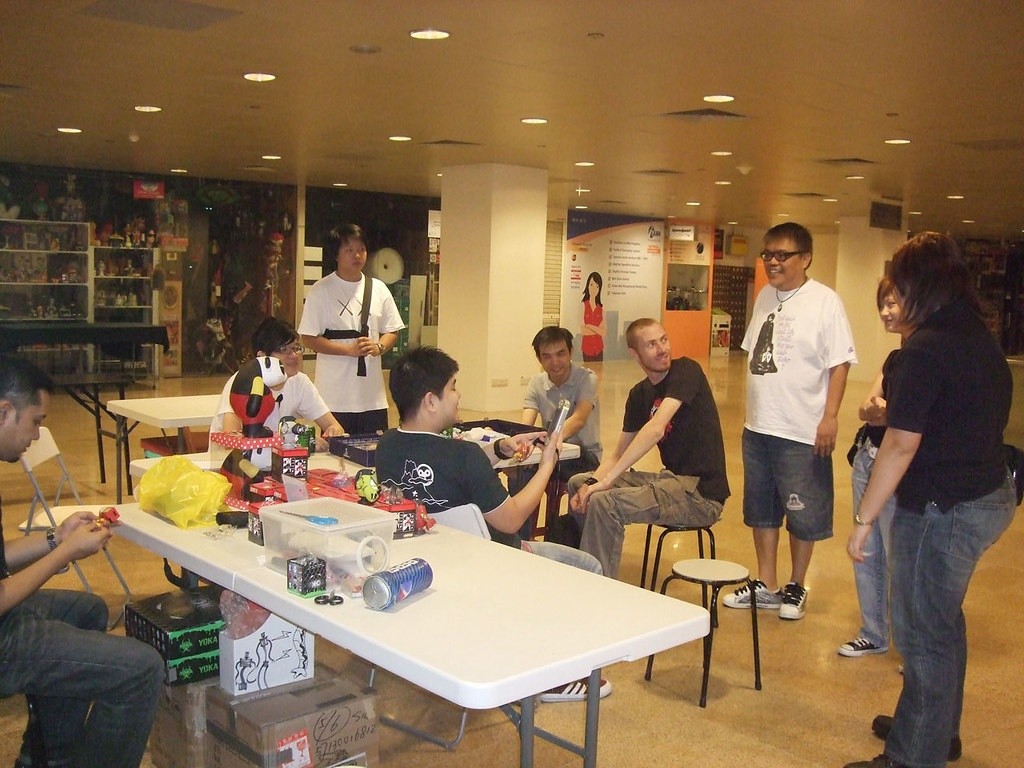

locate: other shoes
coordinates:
[843,753,906,768]
[872,714,961,761]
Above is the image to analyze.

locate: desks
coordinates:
[106,391,223,454]
[0,322,173,496]
[491,439,582,542]
[103,498,309,590]
[130,447,378,592]
[229,511,712,768]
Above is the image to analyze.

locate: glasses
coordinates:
[760,249,807,262]
[269,344,306,355]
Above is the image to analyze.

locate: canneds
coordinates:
[362,558,433,610]
[297,426,316,453]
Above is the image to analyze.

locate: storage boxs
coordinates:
[218,611,315,697]
[150,704,207,768]
[157,674,222,732]
[162,647,220,689]
[206,660,382,768]
[202,730,256,768]
[124,583,226,662]
[258,496,399,593]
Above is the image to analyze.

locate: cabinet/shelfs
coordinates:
[0,218,160,391]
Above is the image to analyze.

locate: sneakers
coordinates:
[838,636,889,656]
[779,582,808,618]
[723,580,782,608]
[541,679,612,703]
[898,663,903,674]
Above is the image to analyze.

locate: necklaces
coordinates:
[775,276,807,312]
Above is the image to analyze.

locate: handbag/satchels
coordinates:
[847,423,871,467]
[139,456,233,529]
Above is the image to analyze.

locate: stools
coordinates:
[641,558,762,709]
[640,517,722,610]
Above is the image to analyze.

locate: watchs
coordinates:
[46,526,59,550]
[852,513,875,526]
[376,342,385,356]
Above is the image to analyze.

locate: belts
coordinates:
[865,437,878,459]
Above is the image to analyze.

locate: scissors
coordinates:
[315,591,344,605]
[280,510,338,525]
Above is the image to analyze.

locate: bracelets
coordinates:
[494,438,512,460]
[583,476,598,485]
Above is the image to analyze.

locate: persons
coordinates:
[721,223,857,617]
[837,275,920,672]
[505,326,603,540]
[844,231,1016,768]
[374,344,612,702]
[297,223,406,436]
[568,318,731,579]
[0,353,163,768]
[207,317,344,452]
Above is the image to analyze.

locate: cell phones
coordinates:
[544,400,569,444]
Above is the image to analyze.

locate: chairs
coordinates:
[369,502,523,748]
[18,424,136,632]
